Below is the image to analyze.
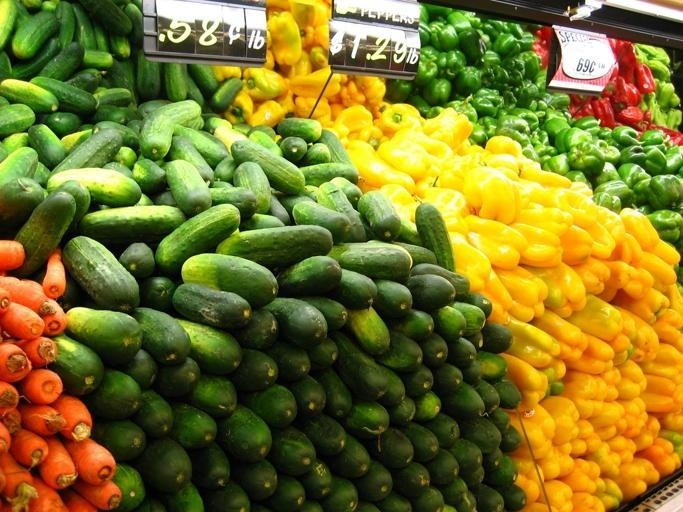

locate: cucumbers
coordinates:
[0,0,527,512]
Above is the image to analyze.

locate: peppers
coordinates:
[212,0,683,512]
